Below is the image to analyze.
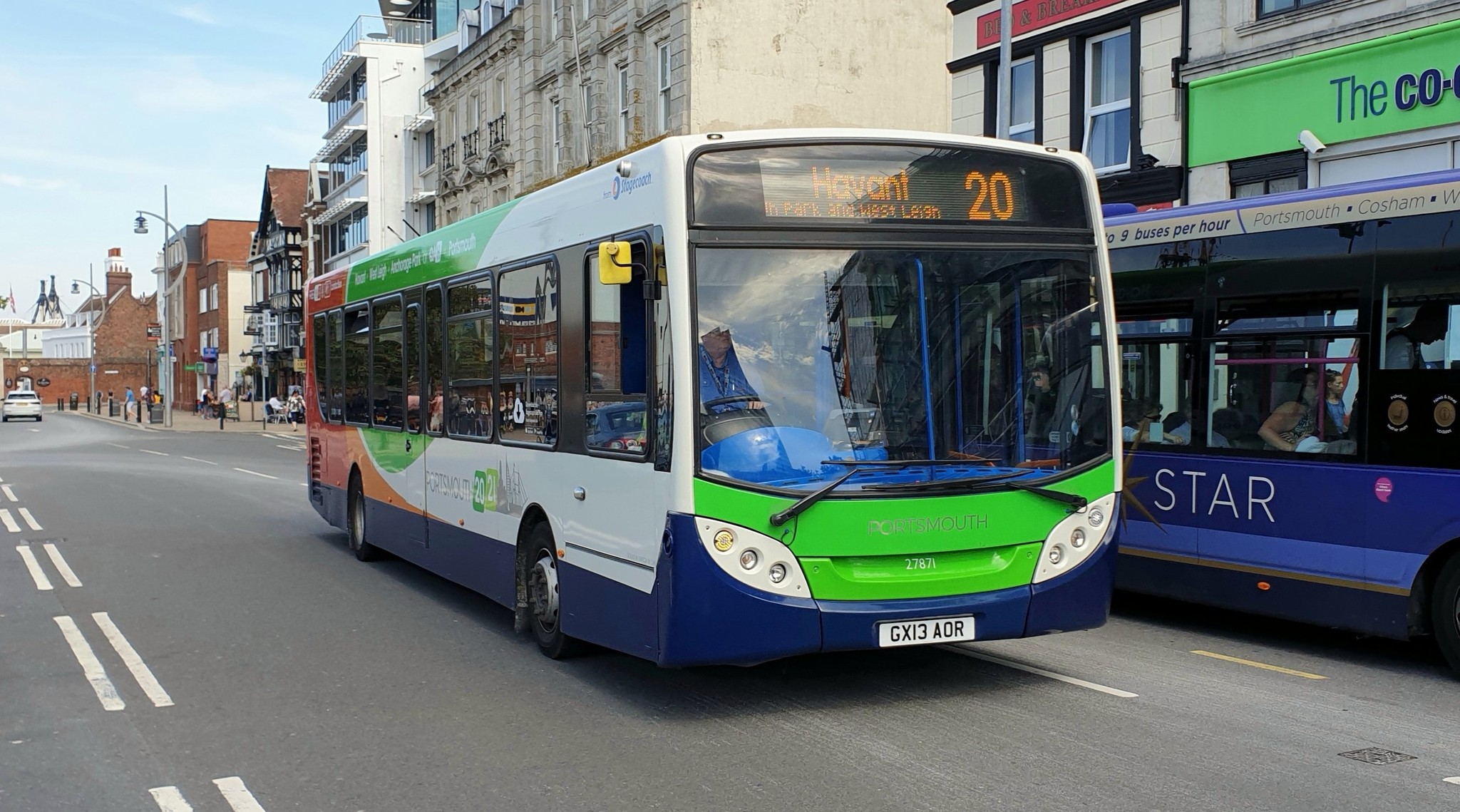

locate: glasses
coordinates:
[1216,422,1241,430]
[709,328,731,336]
[1143,415,1161,422]
[1303,383,1317,389]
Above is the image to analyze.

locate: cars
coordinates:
[0,390,43,422]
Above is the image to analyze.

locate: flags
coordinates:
[10,286,16,313]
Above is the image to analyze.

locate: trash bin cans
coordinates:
[69,392,78,410]
[152,403,163,424]
[112,400,121,416]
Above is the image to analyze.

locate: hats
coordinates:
[700,319,730,337]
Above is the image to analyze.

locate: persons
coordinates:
[1160,397,1231,448]
[1256,366,1320,451]
[122,385,142,421]
[237,387,252,403]
[1325,368,1349,437]
[428,390,443,433]
[151,390,161,405]
[697,319,771,417]
[588,401,611,410]
[285,389,307,431]
[1385,300,1448,369]
[334,388,343,418]
[95,388,114,410]
[200,385,233,420]
[1121,380,1132,401]
[140,383,151,403]
[1121,396,1184,445]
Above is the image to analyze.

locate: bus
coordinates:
[304,126,1125,672]
[952,166,1460,674]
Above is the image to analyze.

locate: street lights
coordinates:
[134,184,188,428]
[70,263,105,414]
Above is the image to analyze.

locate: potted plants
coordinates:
[239,362,256,377]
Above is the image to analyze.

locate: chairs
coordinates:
[265,403,306,424]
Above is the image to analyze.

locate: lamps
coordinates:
[266,354,275,374]
[239,349,247,363]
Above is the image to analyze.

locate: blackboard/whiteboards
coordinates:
[224,401,239,419]
